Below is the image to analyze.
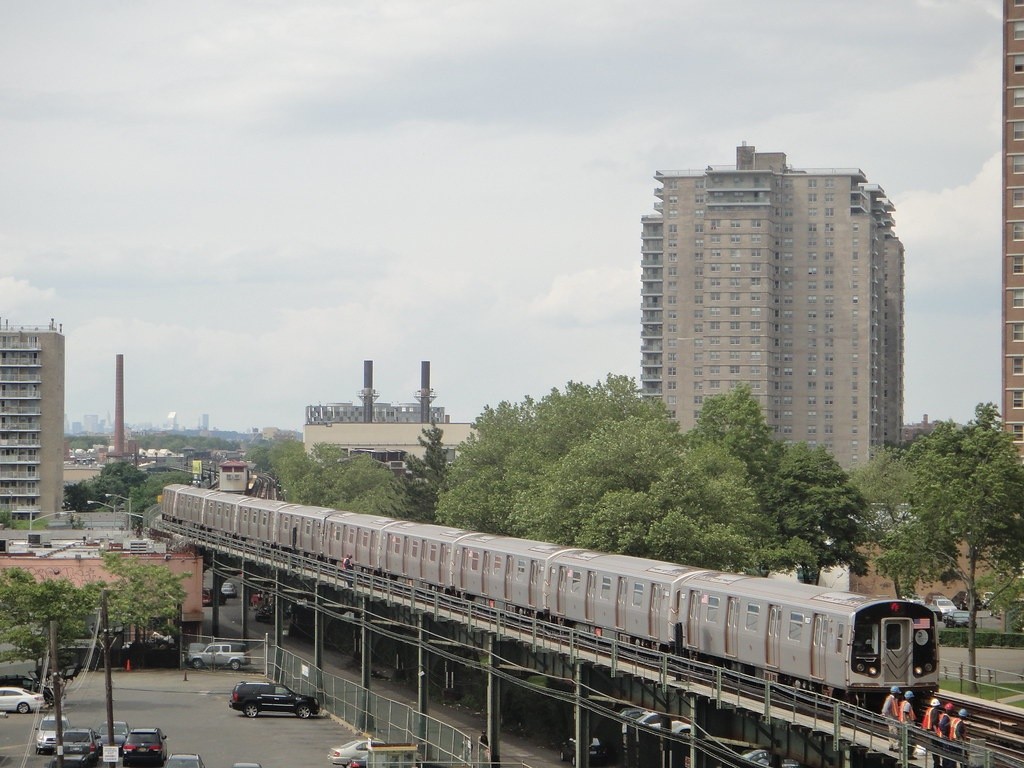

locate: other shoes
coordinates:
[934,765,943,768]
[348,585,353,587]
[908,754,918,760]
[889,748,903,753]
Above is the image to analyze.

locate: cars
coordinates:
[201,582,238,605]
[164,753,207,768]
[0,687,47,714]
[182,642,206,661]
[906,592,978,629]
[232,762,263,768]
[954,590,1004,619]
[559,734,611,768]
[98,720,129,754]
[326,739,391,768]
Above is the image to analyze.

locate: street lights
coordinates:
[105,493,133,537]
[87,500,117,529]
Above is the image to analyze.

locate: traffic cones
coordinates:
[127,659,132,671]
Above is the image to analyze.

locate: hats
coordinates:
[347,553,353,558]
[891,685,900,694]
[959,708,967,716]
[945,703,955,710]
[931,698,940,706]
[905,691,914,698]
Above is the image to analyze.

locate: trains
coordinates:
[159,483,941,709]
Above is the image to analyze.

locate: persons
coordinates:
[344,553,353,588]
[898,690,919,760]
[922,698,942,768]
[949,708,969,768]
[480,730,487,746]
[937,703,955,768]
[881,686,903,752]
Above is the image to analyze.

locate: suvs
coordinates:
[36,714,69,755]
[228,681,321,719]
[188,641,251,671]
[49,726,102,768]
[123,727,168,766]
